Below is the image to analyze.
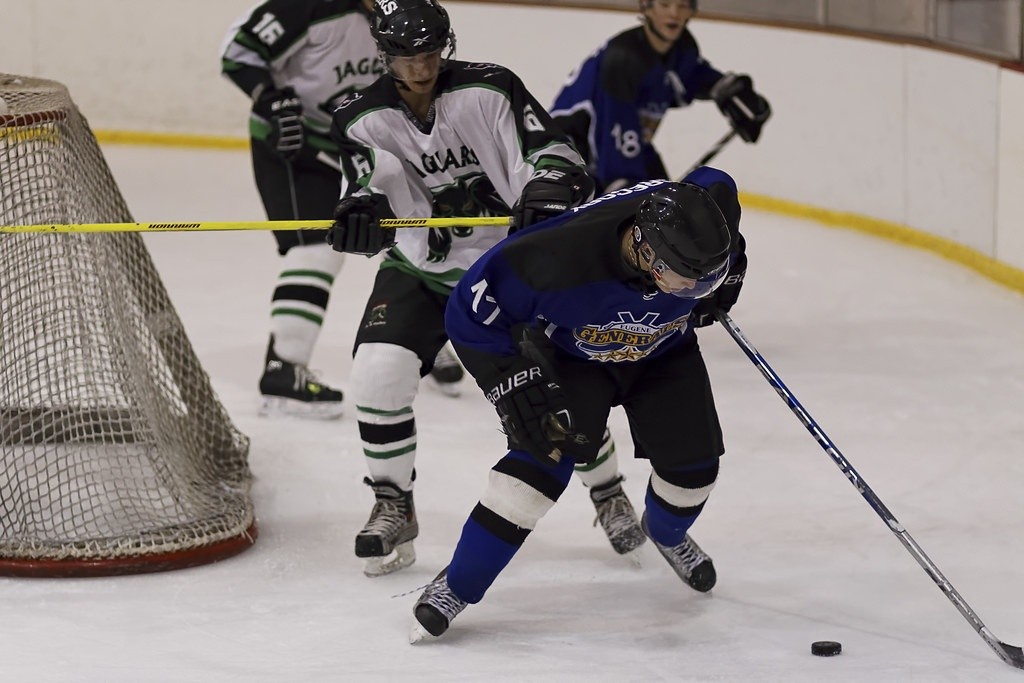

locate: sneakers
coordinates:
[260,364,345,421]
[426,345,465,398]
[408,564,468,644]
[640,509,717,594]
[590,474,648,555]
[355,468,419,577]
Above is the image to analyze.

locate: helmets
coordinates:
[369,0,451,56]
[635,183,731,280]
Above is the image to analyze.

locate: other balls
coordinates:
[810,640,843,656]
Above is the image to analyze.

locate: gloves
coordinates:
[689,298,725,328]
[472,354,577,466]
[516,167,577,232]
[325,194,398,253]
[252,86,306,160]
[712,71,771,144]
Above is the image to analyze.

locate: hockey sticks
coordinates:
[299,141,344,176]
[697,294,1024,672]
[2,213,516,237]
[672,126,738,186]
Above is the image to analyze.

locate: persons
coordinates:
[220,0,465,423]
[409,165,749,645]
[352,0,649,579]
[547,0,772,199]
